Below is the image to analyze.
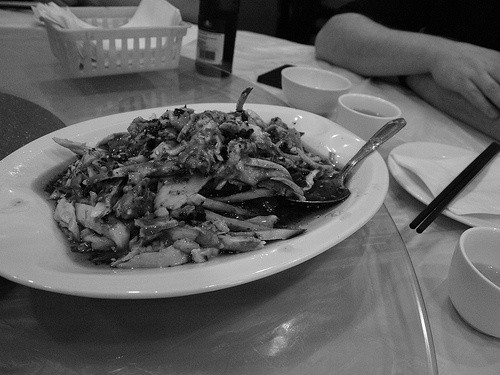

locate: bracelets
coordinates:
[396,72,411,90]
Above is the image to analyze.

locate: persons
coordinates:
[314,0,500,142]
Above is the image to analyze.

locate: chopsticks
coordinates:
[409,139,499,233]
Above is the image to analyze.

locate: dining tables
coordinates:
[0,8,500,375]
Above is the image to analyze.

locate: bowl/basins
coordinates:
[281,67,352,114]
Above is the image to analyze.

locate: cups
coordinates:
[335,94,402,141]
[447,227,500,341]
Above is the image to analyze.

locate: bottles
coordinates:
[195,0,239,77]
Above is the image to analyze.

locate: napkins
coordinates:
[392,154,500,216]
[29,0,182,67]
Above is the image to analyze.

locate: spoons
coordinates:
[282,117,407,205]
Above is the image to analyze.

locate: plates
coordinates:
[1,102,389,300]
[387,141,500,229]
